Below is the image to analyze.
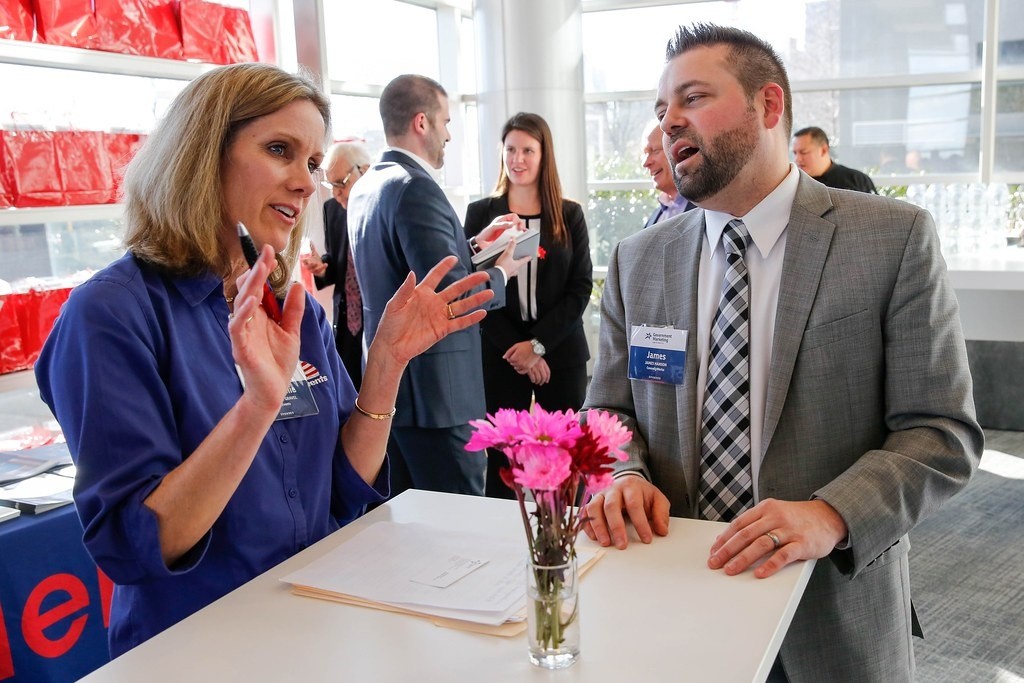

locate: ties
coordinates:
[346,243,361,335]
[698,218,755,524]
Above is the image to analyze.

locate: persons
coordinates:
[642,124,698,228]
[347,74,532,497]
[303,143,372,394]
[792,127,878,195]
[578,23,984,683]
[464,113,593,502]
[35,63,494,662]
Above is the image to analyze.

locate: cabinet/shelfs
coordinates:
[0,0,298,375]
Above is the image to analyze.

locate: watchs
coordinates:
[531,338,545,356]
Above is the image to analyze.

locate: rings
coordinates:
[766,532,779,549]
[449,304,455,319]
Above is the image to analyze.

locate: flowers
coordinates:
[463,400,635,655]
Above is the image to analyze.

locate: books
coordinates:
[471,228,540,272]
[0,441,76,523]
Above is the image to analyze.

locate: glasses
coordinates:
[319,165,355,189]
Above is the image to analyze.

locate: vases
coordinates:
[525,556,583,670]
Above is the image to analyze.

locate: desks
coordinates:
[71,487,820,683]
[0,463,117,683]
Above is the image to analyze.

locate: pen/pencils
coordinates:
[236,220,281,326]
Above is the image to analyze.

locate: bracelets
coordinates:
[470,236,482,254]
[355,397,396,420]
